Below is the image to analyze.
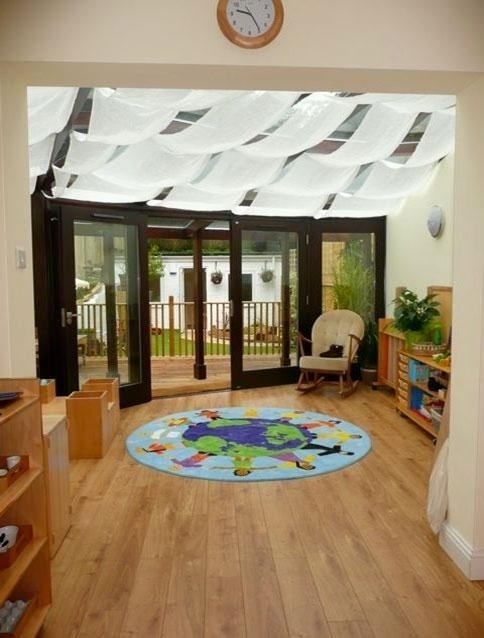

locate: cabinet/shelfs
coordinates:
[0,378,70,638]
[395,350,450,444]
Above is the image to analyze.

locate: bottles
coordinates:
[433,324,443,345]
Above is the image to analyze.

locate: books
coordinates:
[413,363,429,383]
[438,374,448,386]
[409,359,423,383]
[419,400,444,419]
[429,406,443,416]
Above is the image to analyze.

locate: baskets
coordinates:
[412,340,449,357]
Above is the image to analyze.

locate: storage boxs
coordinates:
[430,408,443,434]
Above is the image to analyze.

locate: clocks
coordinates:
[217,0,284,50]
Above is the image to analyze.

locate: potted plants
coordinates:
[383,289,448,355]
[210,271,223,284]
[260,271,273,283]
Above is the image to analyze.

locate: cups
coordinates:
[7,456,21,474]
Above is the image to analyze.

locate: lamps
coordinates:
[427,204,445,240]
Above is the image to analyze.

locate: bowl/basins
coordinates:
[0,525,20,553]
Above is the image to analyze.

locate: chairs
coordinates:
[294,309,365,397]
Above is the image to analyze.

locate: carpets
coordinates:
[125,407,373,482]
